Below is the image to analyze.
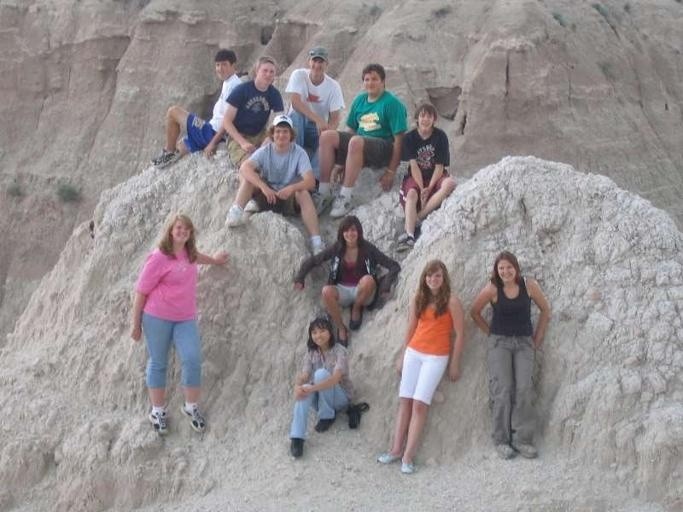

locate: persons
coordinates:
[311,62,408,218]
[149,48,241,167]
[469,249,551,460]
[378,259,465,473]
[293,214,401,350]
[227,114,323,257]
[128,215,231,437]
[398,103,454,247]
[223,55,283,169]
[287,316,353,459]
[284,47,346,182]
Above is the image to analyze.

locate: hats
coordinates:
[307,47,329,64]
[271,115,294,130]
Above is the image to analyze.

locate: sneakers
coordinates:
[335,323,348,347]
[492,438,515,460]
[179,400,206,433]
[149,147,180,169]
[309,241,325,257]
[290,438,303,457]
[328,190,358,217]
[148,410,168,436]
[400,453,417,475]
[314,409,335,432]
[510,439,537,458]
[349,305,362,329]
[310,182,337,215]
[377,447,403,464]
[245,198,260,213]
[394,221,421,247]
[224,204,244,226]
